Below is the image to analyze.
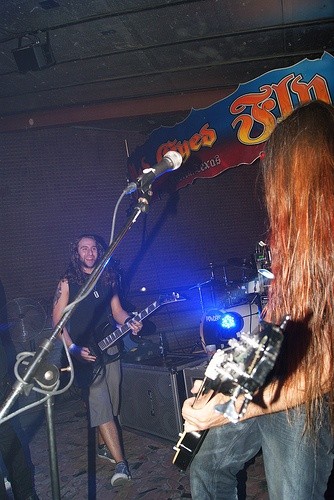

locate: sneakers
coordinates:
[111,462,132,486]
[97,445,116,463]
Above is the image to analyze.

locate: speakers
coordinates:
[116,350,210,446]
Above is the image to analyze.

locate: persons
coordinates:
[51,233,143,486]
[183,99,334,500]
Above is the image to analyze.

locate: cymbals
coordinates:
[196,263,223,270]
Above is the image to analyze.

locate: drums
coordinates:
[199,303,261,354]
[244,281,260,294]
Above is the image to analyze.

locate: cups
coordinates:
[205,344,216,363]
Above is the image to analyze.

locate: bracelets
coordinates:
[68,344,80,357]
[124,317,132,324]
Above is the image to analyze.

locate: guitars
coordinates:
[171,314,292,472]
[61,292,187,388]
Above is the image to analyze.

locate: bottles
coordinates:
[158,333,165,357]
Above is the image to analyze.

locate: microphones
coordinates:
[125,151,183,195]
